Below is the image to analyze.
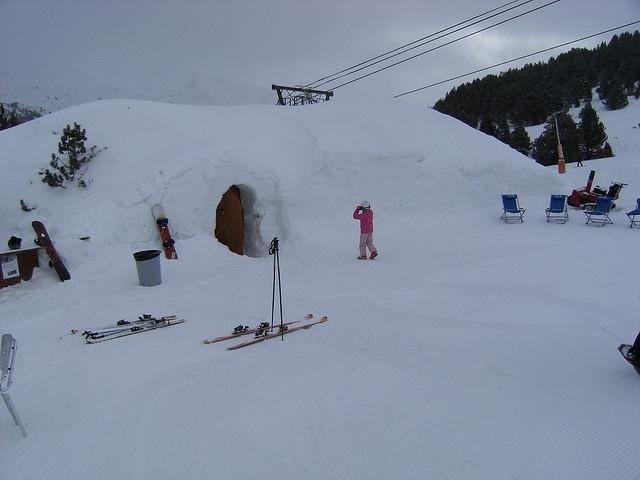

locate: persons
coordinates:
[577,155,583,167]
[353,202,378,260]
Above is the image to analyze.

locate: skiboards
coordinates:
[203,314,328,351]
[82,315,185,345]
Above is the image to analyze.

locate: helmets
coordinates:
[361,200,370,208]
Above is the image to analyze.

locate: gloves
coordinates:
[357,206,364,210]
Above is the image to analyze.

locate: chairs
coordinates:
[583,195,613,228]
[544,193,569,224]
[0,333,28,438]
[500,193,525,223]
[626,198,640,228]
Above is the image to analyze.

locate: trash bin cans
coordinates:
[133,250,162,287]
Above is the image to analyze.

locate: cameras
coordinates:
[357,205,362,211]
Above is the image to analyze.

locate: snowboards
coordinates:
[33,222,72,283]
[152,204,178,260]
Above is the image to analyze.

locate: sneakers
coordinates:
[357,251,378,260]
